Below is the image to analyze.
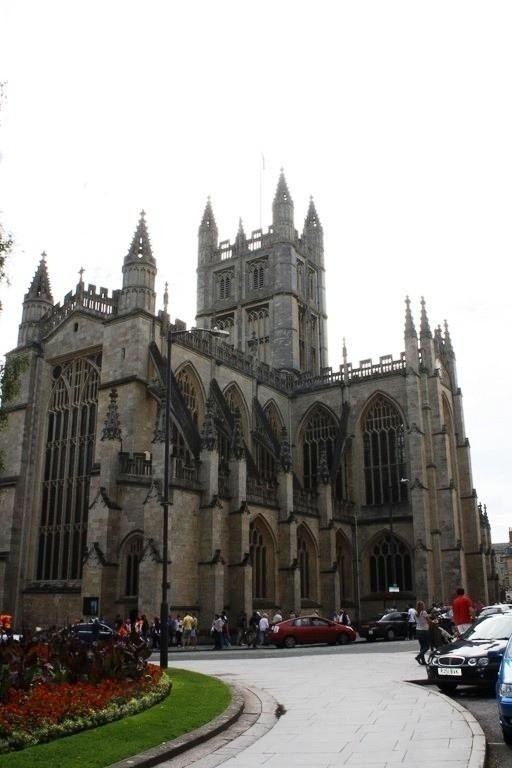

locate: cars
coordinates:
[55,622,153,663]
[475,603,512,620]
[358,611,416,642]
[264,614,356,648]
[495,632,512,743]
[427,612,511,693]
[432,608,455,636]
[0,625,23,642]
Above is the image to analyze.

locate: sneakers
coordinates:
[421,663,429,665]
[415,657,420,664]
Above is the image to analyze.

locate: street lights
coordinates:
[388,477,413,610]
[159,326,231,667]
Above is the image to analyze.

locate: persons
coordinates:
[91,609,351,652]
[384,587,485,666]
